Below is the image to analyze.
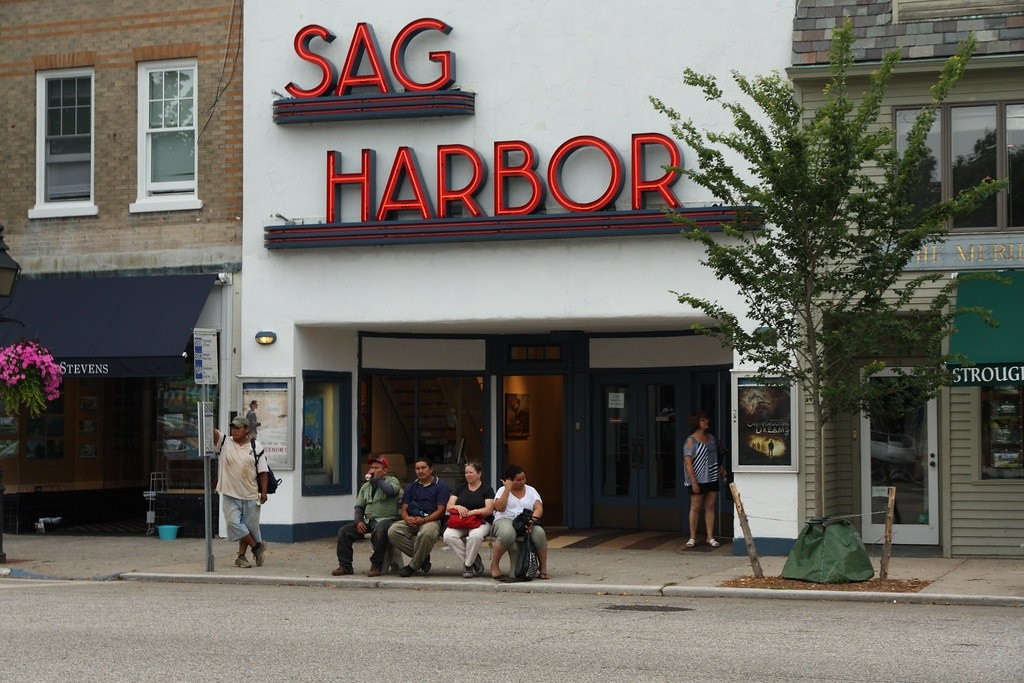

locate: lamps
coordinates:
[255,331,277,345]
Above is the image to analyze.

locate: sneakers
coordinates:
[368,565,381,577]
[421,554,431,573]
[462,562,473,578]
[333,563,354,575]
[235,552,252,568]
[252,540,266,566]
[472,554,483,575]
[400,565,415,577]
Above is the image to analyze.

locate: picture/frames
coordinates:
[304,391,326,475]
[361,373,372,456]
[505,392,530,439]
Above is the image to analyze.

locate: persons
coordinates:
[443,460,495,578]
[246,400,261,457]
[305,435,321,450]
[490,465,551,581]
[682,409,728,548]
[214,416,270,568]
[388,456,451,578]
[332,452,401,581]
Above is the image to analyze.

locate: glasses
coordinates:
[698,416,710,421]
[464,464,477,467]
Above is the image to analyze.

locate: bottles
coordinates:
[365,473,372,481]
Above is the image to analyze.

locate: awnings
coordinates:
[944,270,1024,388]
[0,273,229,378]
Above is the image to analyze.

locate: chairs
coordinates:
[382,453,411,503]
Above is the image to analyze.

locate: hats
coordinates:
[229,416,249,428]
[367,456,387,469]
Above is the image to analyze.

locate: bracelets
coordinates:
[423,518,425,523]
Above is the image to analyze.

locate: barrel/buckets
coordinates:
[157,525,179,540]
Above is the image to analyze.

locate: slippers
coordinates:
[706,538,719,547]
[686,539,695,548]
[538,572,549,580]
[496,574,509,581]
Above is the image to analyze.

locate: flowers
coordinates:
[0,333,62,420]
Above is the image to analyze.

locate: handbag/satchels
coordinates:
[513,508,543,536]
[407,505,428,517]
[447,508,487,528]
[515,532,539,581]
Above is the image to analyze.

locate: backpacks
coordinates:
[219,434,276,494]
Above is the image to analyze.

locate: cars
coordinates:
[870,431,922,464]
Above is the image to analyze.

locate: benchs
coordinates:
[364,533,525,579]
